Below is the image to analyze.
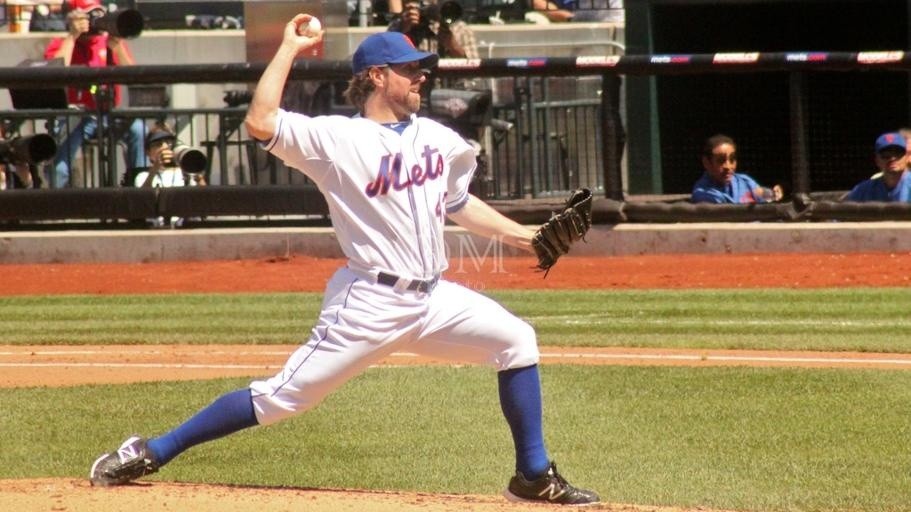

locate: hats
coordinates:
[61,0,107,23]
[350,31,439,78]
[144,128,175,148]
[873,130,906,157]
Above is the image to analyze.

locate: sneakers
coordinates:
[502,460,602,509]
[88,435,159,488]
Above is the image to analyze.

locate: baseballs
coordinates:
[297,15,320,36]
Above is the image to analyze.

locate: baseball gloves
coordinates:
[532,188,593,268]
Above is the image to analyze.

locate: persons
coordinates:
[131,125,208,230]
[89,11,600,508]
[837,127,911,201]
[348,0,577,27]
[41,0,151,188]
[386,0,496,199]
[690,135,785,206]
[0,122,51,227]
[871,123,911,180]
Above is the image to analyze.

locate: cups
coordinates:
[7,4,22,34]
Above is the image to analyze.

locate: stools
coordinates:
[522,131,570,190]
[86,139,128,188]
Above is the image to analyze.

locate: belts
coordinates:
[377,271,441,293]
